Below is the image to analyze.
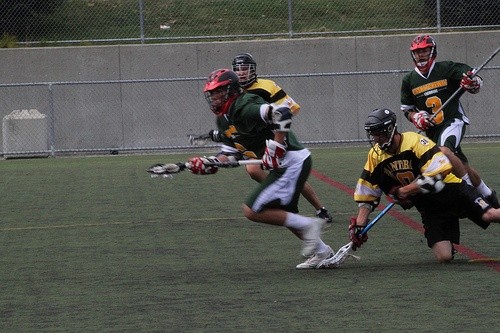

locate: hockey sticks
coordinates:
[317,202,394,270]
[187,134,211,146]
[146,159,262,174]
[417,47,500,134]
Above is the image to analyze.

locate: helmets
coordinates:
[203,69,239,116]
[365,106,396,156]
[410,35,437,73]
[233,53,258,88]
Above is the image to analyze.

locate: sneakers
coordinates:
[302,218,324,257]
[296,246,335,269]
[317,207,332,223]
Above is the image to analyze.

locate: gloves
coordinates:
[348,217,369,251]
[388,186,405,202]
[188,156,220,175]
[261,139,287,170]
[209,128,223,143]
[460,70,480,92]
[413,110,436,131]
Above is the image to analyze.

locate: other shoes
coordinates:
[486,188,499,209]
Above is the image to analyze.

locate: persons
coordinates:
[349,108,500,262]
[208,52,332,223]
[401,34,500,208]
[187,69,335,269]
